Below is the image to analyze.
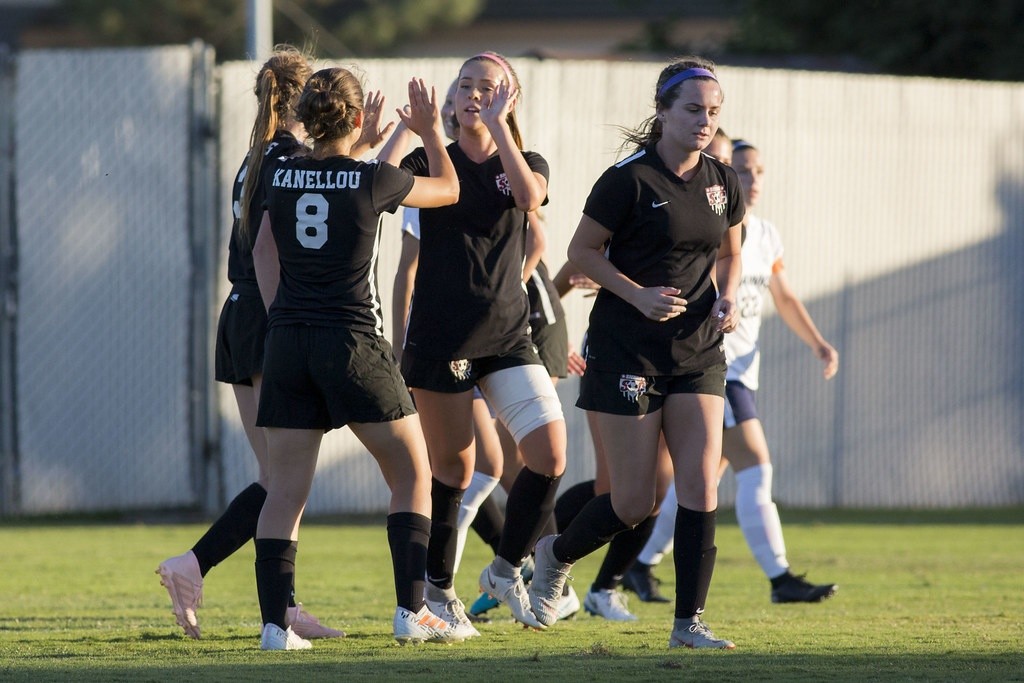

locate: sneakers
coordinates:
[391,603,476,646]
[621,557,674,602]
[479,560,548,631]
[529,534,577,626]
[770,571,838,605]
[425,598,480,636]
[155,549,201,638]
[284,602,346,640]
[470,554,535,616]
[668,611,736,652]
[583,582,639,621]
[263,622,312,649]
[557,584,580,620]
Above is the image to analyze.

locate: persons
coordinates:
[469,260,580,622]
[254,69,475,651]
[527,60,745,650]
[552,129,746,622]
[375,50,567,638]
[392,78,458,363]
[623,139,838,603]
[152,53,346,639]
